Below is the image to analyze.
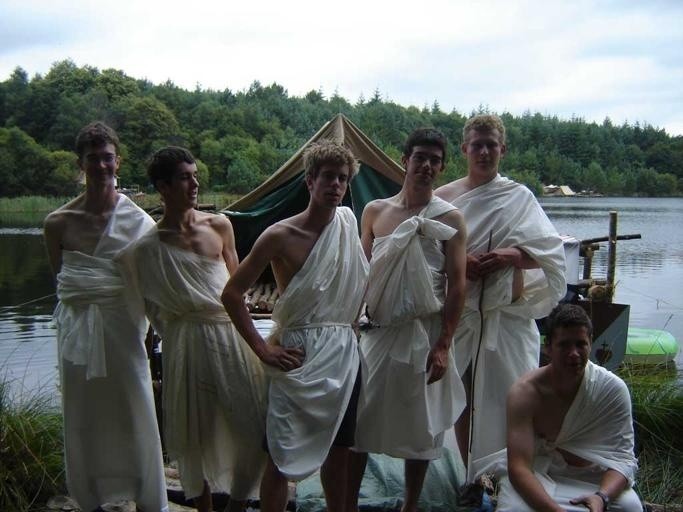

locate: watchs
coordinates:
[595,490,611,512]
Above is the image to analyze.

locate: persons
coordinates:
[431,114,571,512]
[128,147,267,511]
[42,120,156,512]
[493,303,643,512]
[220,138,371,511]
[345,127,469,512]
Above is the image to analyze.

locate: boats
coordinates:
[536,327,678,369]
